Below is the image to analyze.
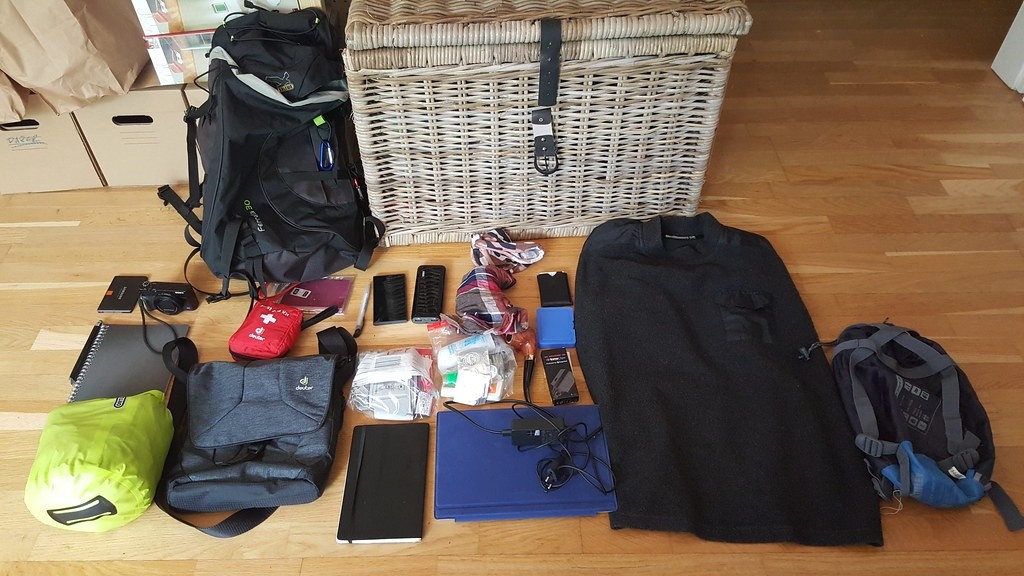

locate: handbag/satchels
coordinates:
[0,0,150,116]
[227,282,302,363]
[0,70,30,126]
[153,324,358,539]
[25,389,175,533]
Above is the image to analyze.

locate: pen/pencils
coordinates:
[353,283,371,337]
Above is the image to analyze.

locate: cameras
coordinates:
[141,281,198,315]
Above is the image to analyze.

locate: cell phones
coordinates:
[373,273,408,326]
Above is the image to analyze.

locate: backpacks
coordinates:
[157,1,389,320]
[828,320,1023,531]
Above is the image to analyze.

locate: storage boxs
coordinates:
[131,0,300,37]
[0,92,108,196]
[72,83,205,188]
[143,28,216,86]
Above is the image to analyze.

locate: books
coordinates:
[336,422,430,543]
[68,323,189,407]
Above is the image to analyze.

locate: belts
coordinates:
[529,14,562,174]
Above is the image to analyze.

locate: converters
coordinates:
[512,418,566,445]
